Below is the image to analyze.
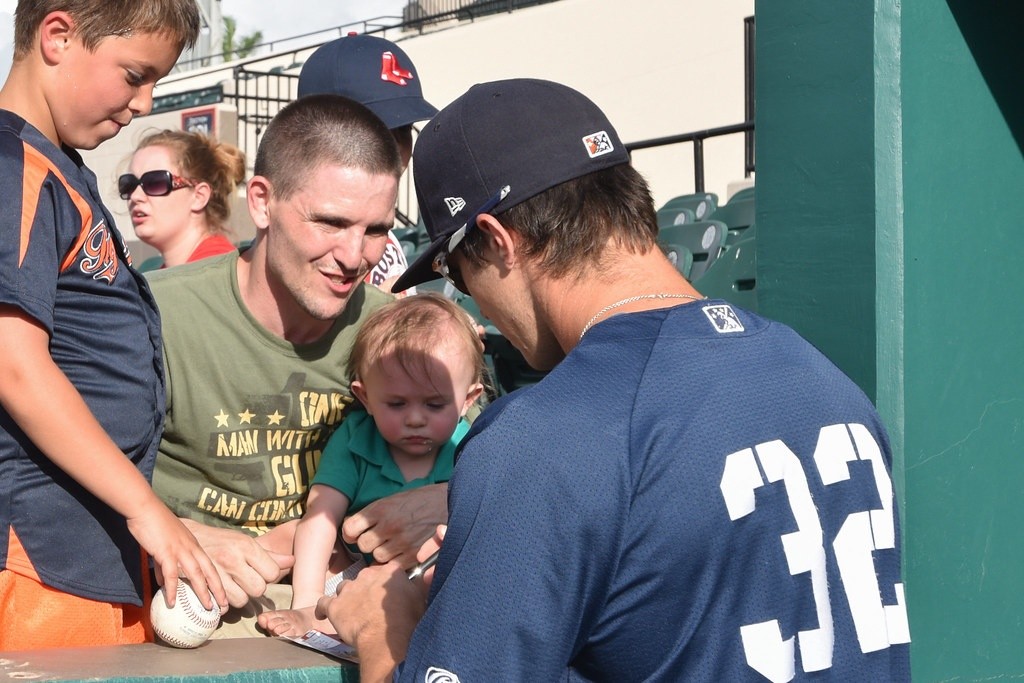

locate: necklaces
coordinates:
[579,294,709,342]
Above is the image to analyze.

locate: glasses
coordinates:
[428,185,511,296]
[118,170,202,200]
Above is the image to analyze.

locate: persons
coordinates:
[0,0,911,682]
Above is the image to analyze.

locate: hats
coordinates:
[298,31,439,130]
[391,79,630,293]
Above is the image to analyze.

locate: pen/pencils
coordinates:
[408,549,440,581]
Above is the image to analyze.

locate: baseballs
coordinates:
[149,575,222,649]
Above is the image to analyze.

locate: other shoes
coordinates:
[150,577,222,649]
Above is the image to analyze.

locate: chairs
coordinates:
[397,188,753,379]
